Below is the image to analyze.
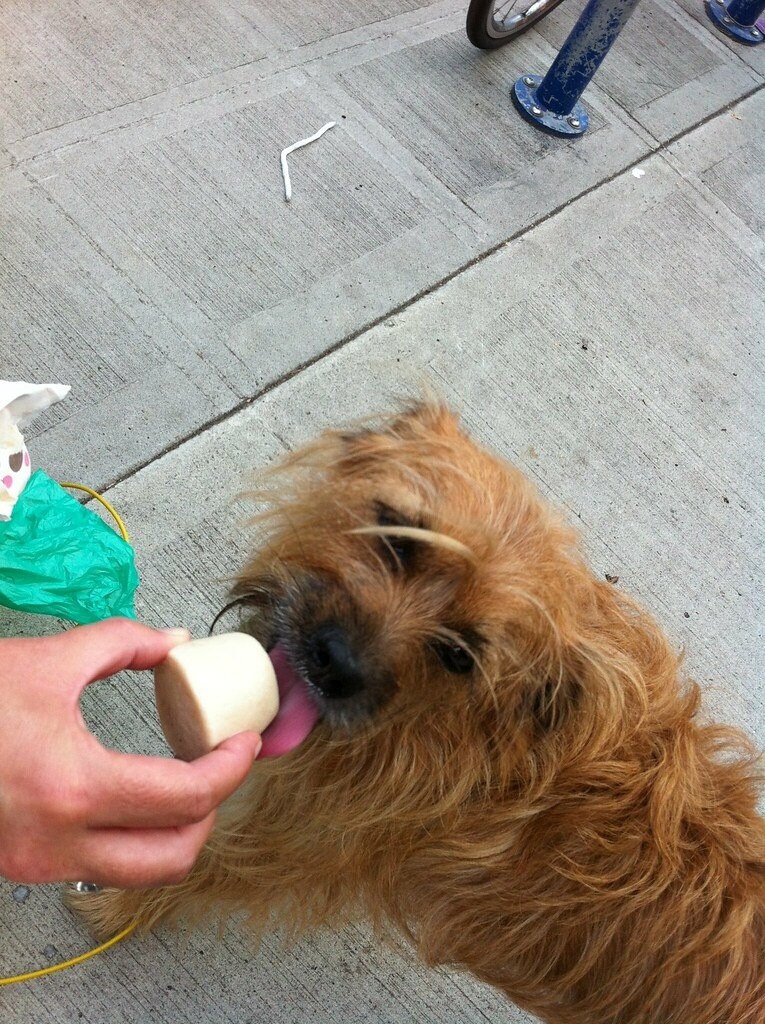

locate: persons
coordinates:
[0,615,263,889]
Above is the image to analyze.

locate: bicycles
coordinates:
[465,0,566,49]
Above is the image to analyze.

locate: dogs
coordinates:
[55,388,765,1024]
